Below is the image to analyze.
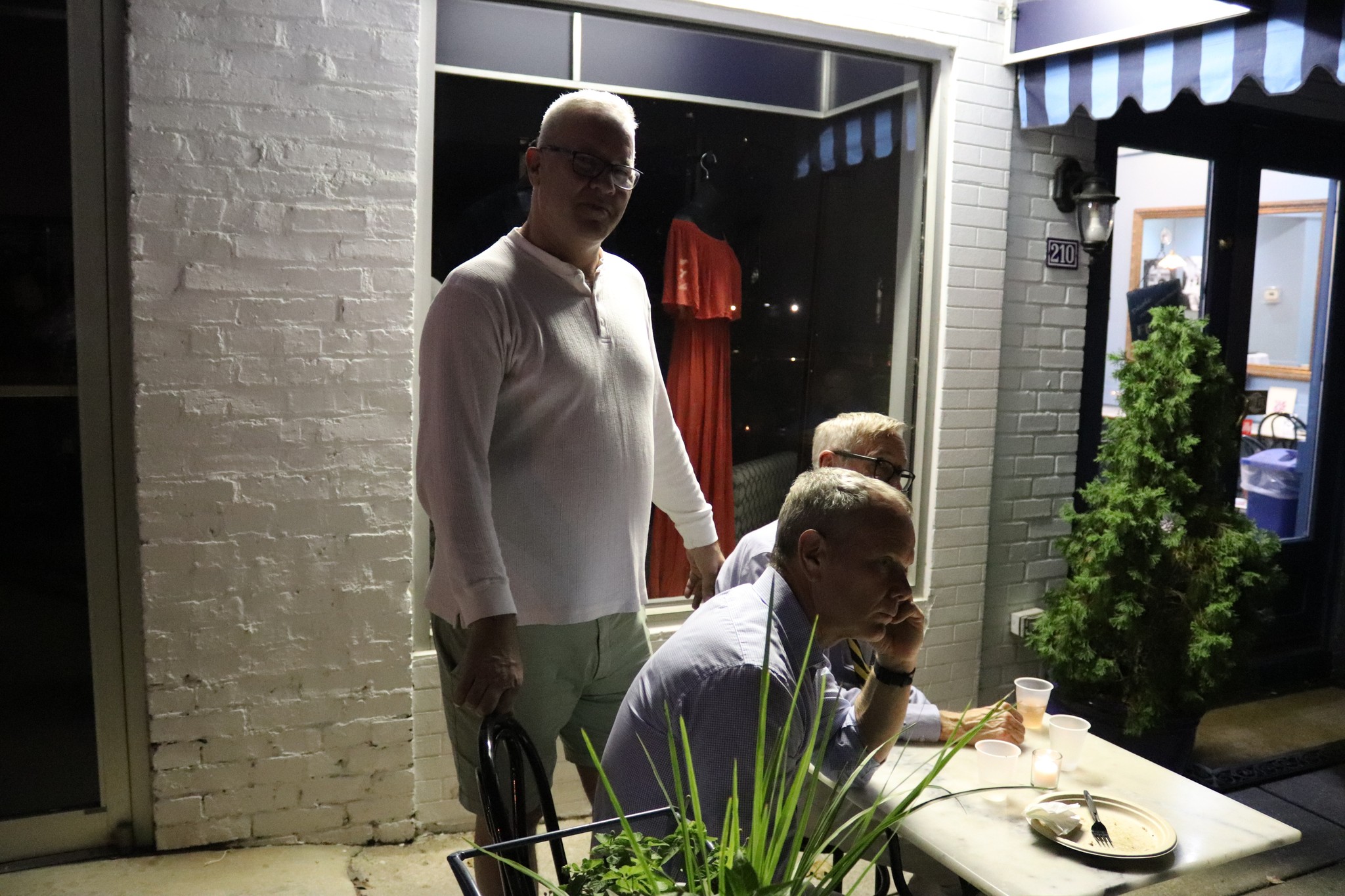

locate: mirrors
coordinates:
[1124,198,1331,380]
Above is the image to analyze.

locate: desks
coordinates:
[838,669,1301,895]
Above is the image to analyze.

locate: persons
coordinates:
[712,411,1025,896]
[419,90,726,896]
[590,465,927,896]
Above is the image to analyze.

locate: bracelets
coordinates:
[872,655,917,687]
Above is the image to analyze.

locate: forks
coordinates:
[1082,790,1113,844]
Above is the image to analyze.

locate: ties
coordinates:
[847,636,871,688]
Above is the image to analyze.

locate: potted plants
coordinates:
[1025,307,1288,777]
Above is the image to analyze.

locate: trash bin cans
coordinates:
[1240,448,1300,538]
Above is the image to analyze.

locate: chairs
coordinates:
[476,699,579,896]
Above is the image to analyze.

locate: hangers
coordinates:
[673,134,732,240]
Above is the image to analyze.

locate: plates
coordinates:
[1025,792,1178,859]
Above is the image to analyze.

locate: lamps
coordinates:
[1053,148,1123,257]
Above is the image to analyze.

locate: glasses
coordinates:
[832,449,916,493]
[539,142,644,191]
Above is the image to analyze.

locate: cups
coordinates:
[1030,749,1063,792]
[974,739,1022,802]
[1047,714,1091,771]
[1014,677,1054,729]
